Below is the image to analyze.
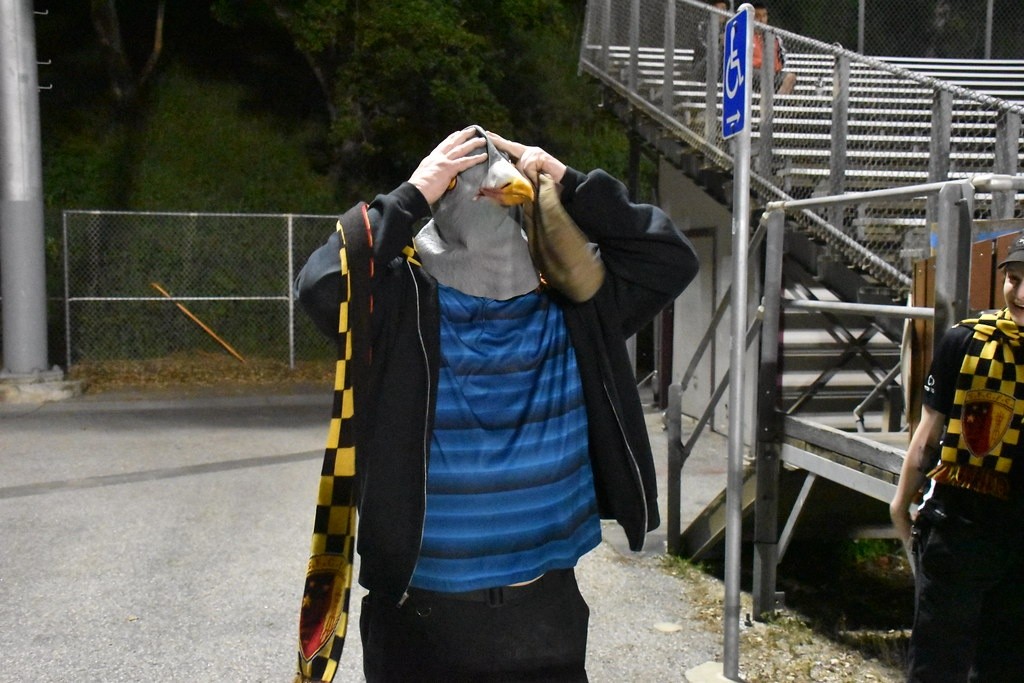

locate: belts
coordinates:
[430,569,561,609]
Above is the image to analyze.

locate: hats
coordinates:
[521,172,603,302]
[998,233,1024,269]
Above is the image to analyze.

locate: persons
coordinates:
[689,0,796,95]
[291,124,699,683]
[888,232,1024,683]
